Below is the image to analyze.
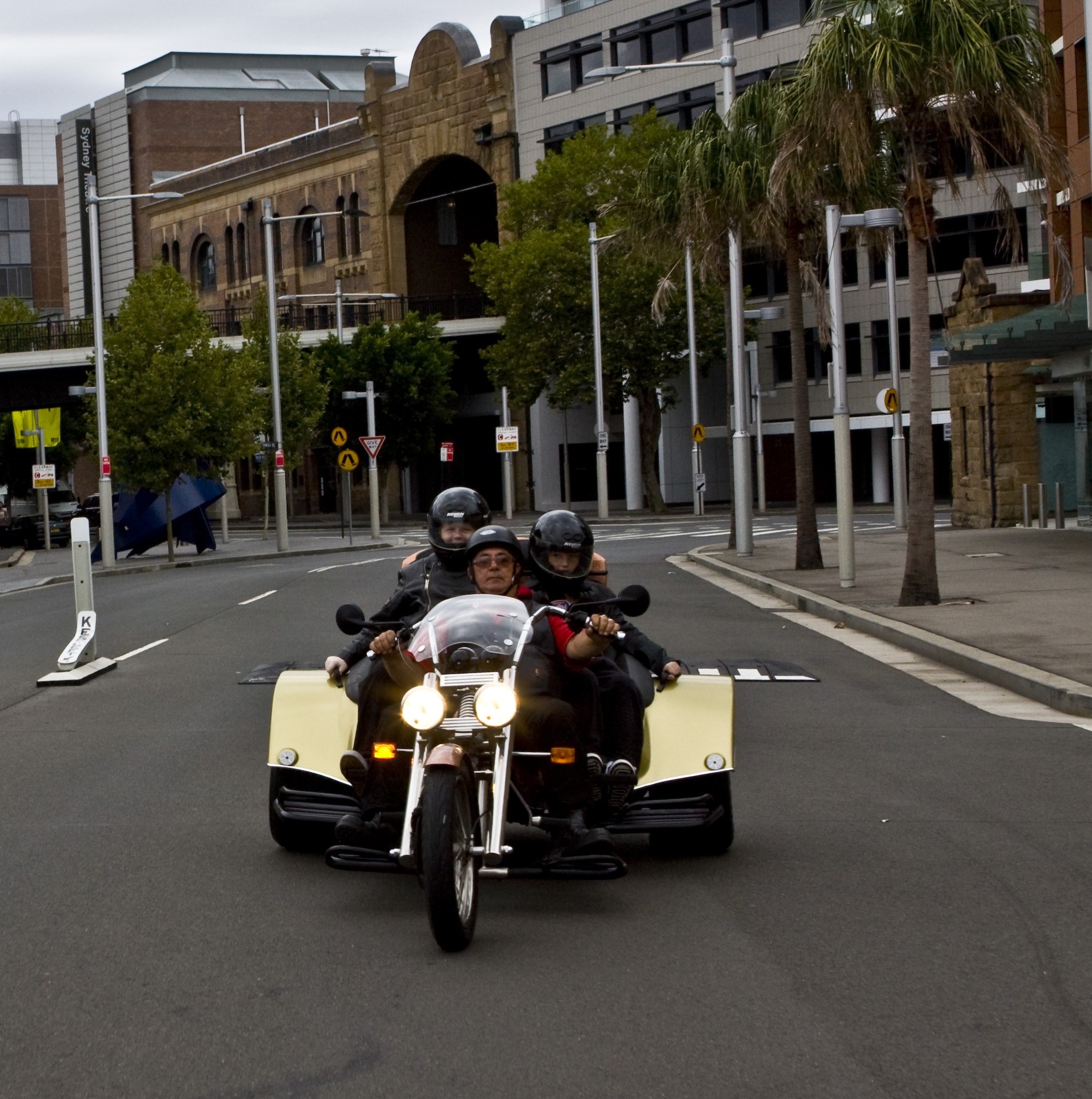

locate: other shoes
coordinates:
[586,753,606,803]
[340,750,368,786]
[606,758,635,806]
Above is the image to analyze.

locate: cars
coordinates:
[0,476,121,551]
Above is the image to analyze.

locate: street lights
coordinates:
[822,204,901,588]
[341,381,381,539]
[277,278,400,527]
[85,172,186,569]
[586,219,618,519]
[259,196,345,552]
[581,27,758,557]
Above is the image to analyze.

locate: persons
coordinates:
[321,490,684,794]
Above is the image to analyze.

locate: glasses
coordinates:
[472,555,513,568]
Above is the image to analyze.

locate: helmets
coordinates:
[427,487,493,562]
[527,510,594,591]
[466,524,521,557]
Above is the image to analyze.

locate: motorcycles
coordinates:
[268,592,736,955]
